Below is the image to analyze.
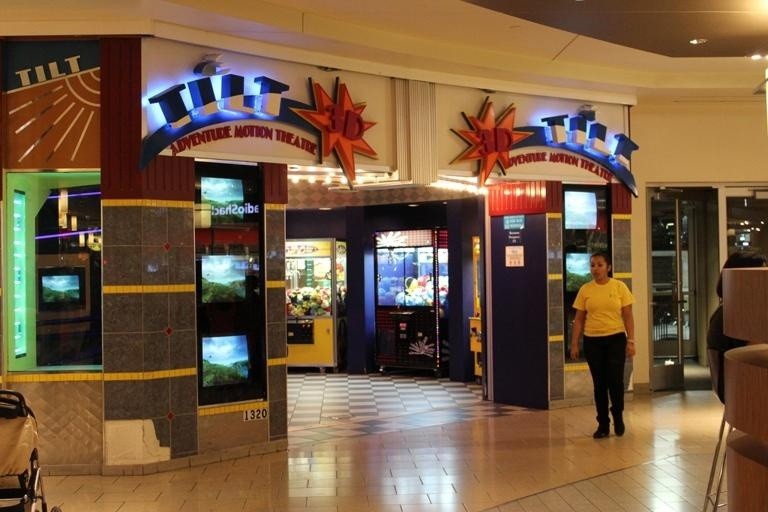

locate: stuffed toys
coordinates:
[395,274,449,308]
[286,264,346,318]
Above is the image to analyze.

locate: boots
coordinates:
[593,415,610,439]
[610,406,624,435]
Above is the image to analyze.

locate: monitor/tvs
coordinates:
[563,190,598,229]
[200,176,245,223]
[202,255,261,304]
[202,334,254,388]
[565,250,593,292]
[37,266,86,310]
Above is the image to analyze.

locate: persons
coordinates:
[706,252,768,405]
[570,252,636,439]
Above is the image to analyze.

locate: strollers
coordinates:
[0,389,61,512]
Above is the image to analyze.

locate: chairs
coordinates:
[698,349,734,512]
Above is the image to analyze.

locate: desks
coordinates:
[653,289,683,323]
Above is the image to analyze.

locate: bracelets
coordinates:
[628,339,636,346]
